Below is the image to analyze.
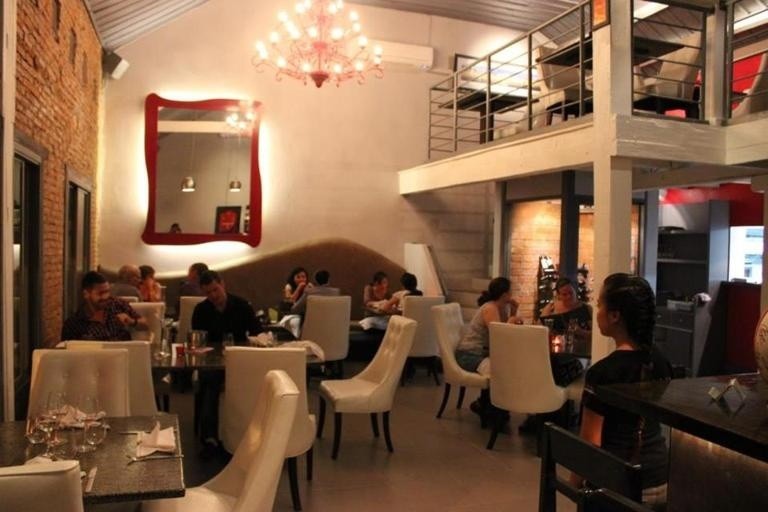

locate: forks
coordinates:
[124,453,186,461]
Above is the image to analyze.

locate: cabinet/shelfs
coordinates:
[655,307,712,377]
[656,225,711,267]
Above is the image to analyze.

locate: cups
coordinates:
[158,318,279,360]
[26,389,109,464]
[515,317,583,345]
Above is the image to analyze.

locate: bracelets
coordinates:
[133,317,137,327]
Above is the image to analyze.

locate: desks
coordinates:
[437,87,539,145]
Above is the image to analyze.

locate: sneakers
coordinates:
[517,415,538,435]
[469,397,485,417]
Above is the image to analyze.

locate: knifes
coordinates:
[117,430,154,436]
[84,466,98,494]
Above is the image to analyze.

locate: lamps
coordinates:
[249,0,386,90]
[182,132,195,194]
[230,132,241,193]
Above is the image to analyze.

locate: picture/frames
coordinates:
[452,52,539,99]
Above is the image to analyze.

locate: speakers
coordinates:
[103,51,131,80]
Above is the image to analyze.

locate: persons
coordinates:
[537,279,592,413]
[383,272,423,377]
[284,267,314,302]
[455,278,523,428]
[566,273,674,512]
[62,272,149,340]
[171,264,208,384]
[137,266,162,302]
[110,265,143,301]
[291,270,340,376]
[192,270,262,462]
[363,272,393,316]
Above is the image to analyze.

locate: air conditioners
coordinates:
[364,38,435,71]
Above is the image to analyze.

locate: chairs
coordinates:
[0,284,597,511]
[534,0,768,128]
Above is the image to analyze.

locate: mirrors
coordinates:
[140,92,264,248]
